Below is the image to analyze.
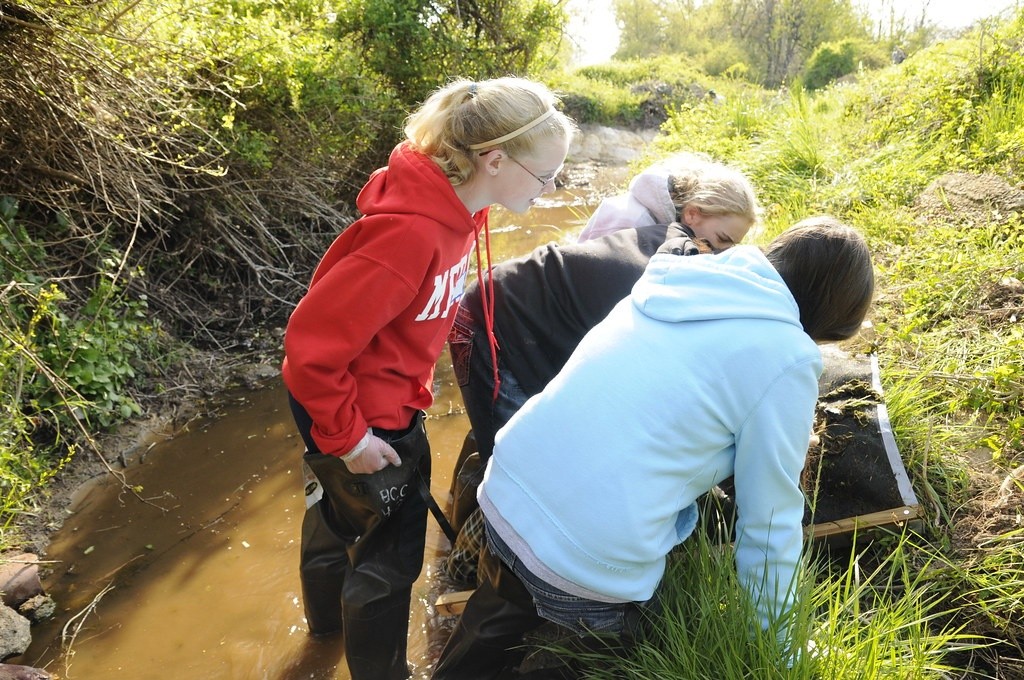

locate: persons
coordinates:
[433,217,875,680]
[281,76,572,680]
[445,150,759,538]
[445,226,728,591]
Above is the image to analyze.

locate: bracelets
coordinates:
[340,435,370,462]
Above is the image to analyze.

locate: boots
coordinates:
[303,562,416,680]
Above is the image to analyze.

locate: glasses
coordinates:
[479,148,565,187]
